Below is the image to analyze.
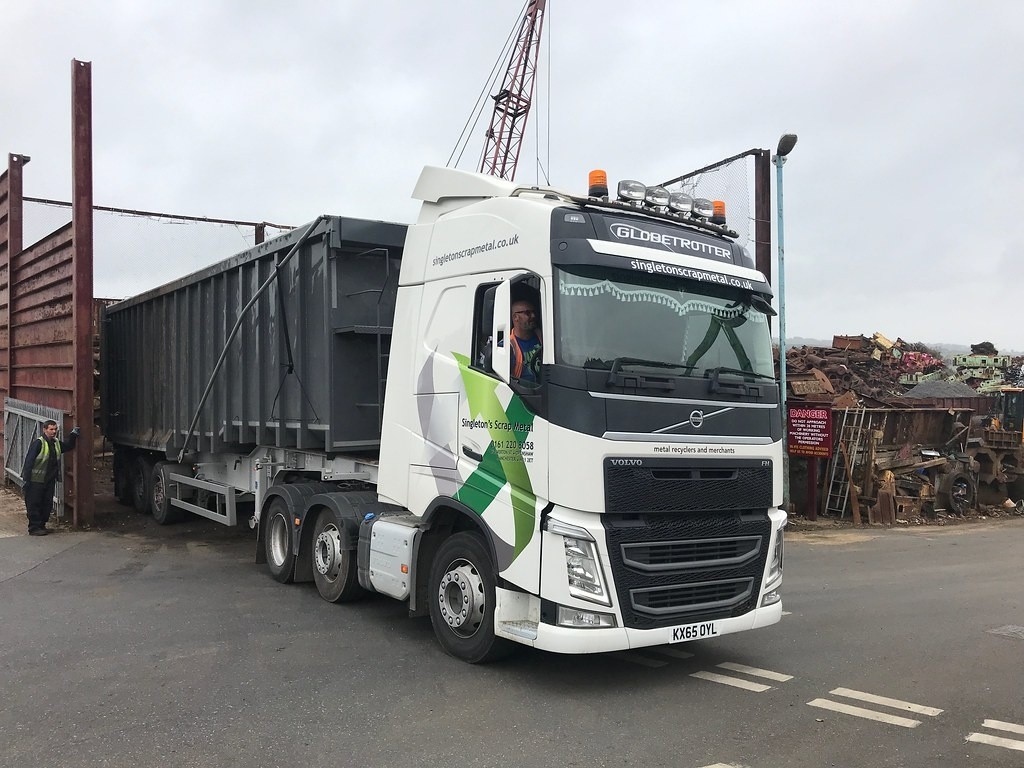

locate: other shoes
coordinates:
[29,529,47,535]
[41,525,54,533]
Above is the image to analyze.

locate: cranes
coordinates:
[445,0,552,186]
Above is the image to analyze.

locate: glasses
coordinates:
[515,310,536,316]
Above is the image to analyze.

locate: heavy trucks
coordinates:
[97,163,789,666]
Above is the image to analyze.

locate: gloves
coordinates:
[72,426,81,436]
[22,481,31,490]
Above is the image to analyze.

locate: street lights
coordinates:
[770,128,799,519]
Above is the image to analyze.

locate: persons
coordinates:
[498,300,543,383]
[23,420,80,536]
